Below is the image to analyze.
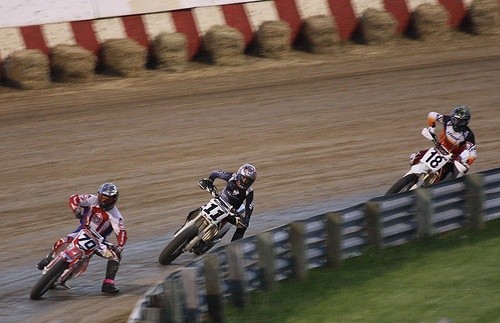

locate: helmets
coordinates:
[450,106,471,131]
[236,163,257,190]
[98,182,119,210]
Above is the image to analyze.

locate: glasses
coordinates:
[452,118,466,126]
[99,195,113,203]
[240,174,253,186]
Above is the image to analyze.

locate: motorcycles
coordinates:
[158,177,249,265]
[383,117,468,196]
[29,209,120,300]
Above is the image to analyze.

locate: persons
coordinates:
[36,182,128,293]
[174,164,257,242]
[410,106,477,181]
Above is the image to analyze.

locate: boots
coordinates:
[102,260,120,294]
[36,250,54,270]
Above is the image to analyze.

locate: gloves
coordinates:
[198,179,213,190]
[239,218,249,228]
